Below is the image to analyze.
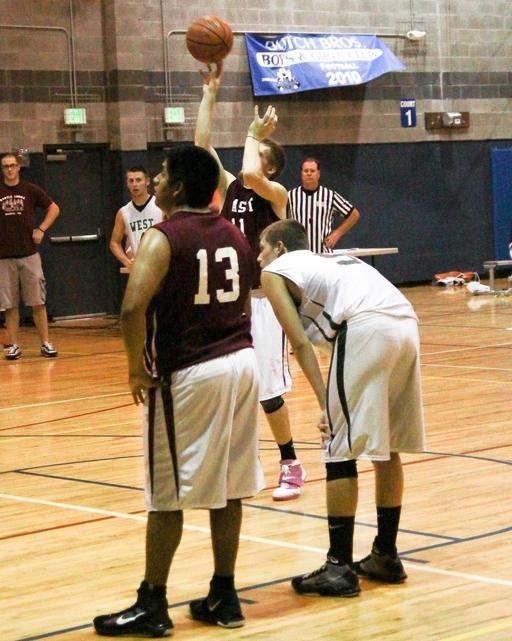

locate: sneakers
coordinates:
[93,601,175,637]
[189,594,247,628]
[272,458,308,500]
[355,543,408,583]
[6,344,23,359]
[291,559,362,598]
[40,341,57,356]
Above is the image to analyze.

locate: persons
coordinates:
[110,164,166,271]
[0,153,60,360]
[92,143,266,638]
[256,219,427,599]
[285,158,360,254]
[195,59,308,501]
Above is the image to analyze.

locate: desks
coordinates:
[332,245,399,270]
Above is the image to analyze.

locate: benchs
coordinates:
[482,259,512,294]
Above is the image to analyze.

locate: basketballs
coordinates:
[186,16,234,63]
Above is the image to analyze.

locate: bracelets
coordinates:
[245,135,261,143]
[38,226,46,233]
